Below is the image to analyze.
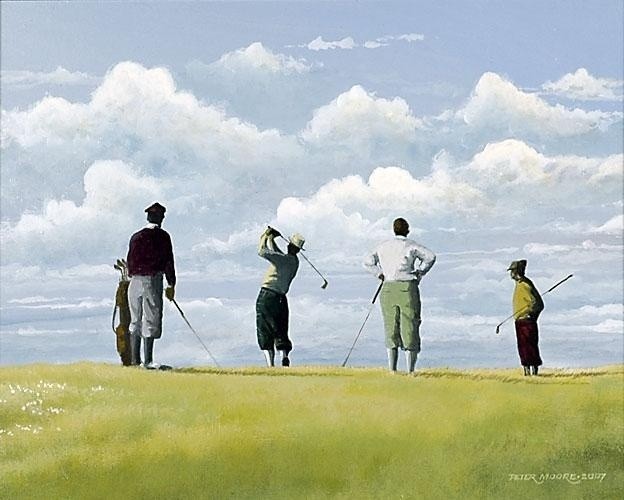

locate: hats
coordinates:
[507,260,527,272]
[144,202,166,220]
[288,231,306,249]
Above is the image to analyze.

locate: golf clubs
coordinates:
[268,225,328,289]
[496,275,572,334]
[114,258,128,281]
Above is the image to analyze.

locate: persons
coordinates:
[124,202,176,370]
[361,217,437,376]
[503,259,544,376]
[254,228,304,367]
